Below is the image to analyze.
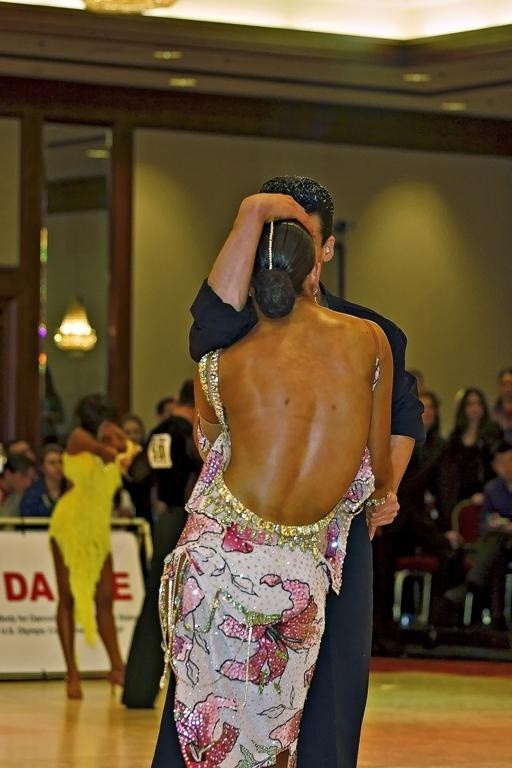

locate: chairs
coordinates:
[393,497,511,626]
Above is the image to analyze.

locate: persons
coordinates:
[157,215,395,767]
[0,440,65,530]
[117,377,204,716]
[119,397,173,531]
[48,388,144,703]
[372,369,512,658]
[148,172,428,768]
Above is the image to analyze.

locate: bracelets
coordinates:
[366,489,393,507]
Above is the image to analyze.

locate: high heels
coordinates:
[64,670,124,700]
